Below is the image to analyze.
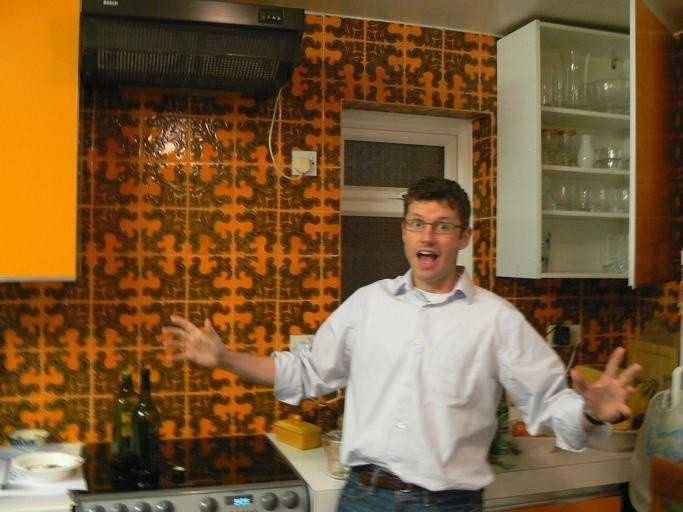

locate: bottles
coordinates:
[489,386,509,456]
[541,231,551,274]
[541,129,628,169]
[130,367,160,475]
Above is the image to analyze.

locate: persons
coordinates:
[158,173,645,511]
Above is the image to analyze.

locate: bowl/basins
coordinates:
[586,424,637,453]
[511,434,559,457]
[578,77,630,114]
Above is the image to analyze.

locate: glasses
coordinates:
[399,217,463,234]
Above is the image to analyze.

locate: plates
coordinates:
[4,428,49,450]
[12,451,84,482]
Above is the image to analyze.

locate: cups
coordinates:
[598,233,624,273]
[112,370,139,461]
[540,47,591,110]
[321,430,352,480]
[544,181,629,215]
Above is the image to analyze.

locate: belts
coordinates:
[358,469,420,494]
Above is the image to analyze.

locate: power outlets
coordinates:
[292,150,318,177]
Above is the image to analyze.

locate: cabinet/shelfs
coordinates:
[495,1,682,291]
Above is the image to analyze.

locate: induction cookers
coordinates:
[71,434,310,512]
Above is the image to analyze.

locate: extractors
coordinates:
[77,0,306,101]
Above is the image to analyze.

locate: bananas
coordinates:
[571,365,658,432]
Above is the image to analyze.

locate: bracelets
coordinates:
[582,406,607,427]
